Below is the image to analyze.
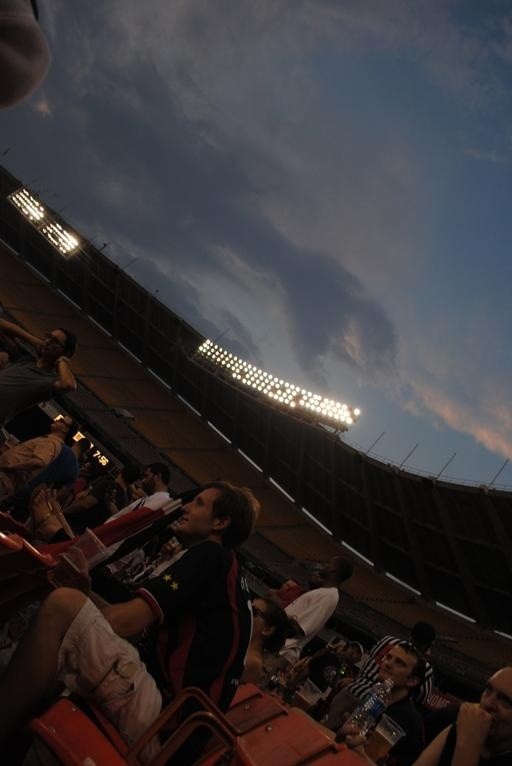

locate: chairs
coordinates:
[0,235,512,766]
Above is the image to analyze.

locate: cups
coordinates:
[363,714,406,763]
[290,679,323,712]
[48,527,109,588]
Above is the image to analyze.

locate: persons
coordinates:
[1,314,82,405]
[2,406,512,766]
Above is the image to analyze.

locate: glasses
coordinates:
[252,605,266,619]
[59,418,69,428]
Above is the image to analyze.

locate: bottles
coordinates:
[341,677,395,744]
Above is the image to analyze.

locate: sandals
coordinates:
[28,481,65,542]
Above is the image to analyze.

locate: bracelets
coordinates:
[57,356,73,369]
